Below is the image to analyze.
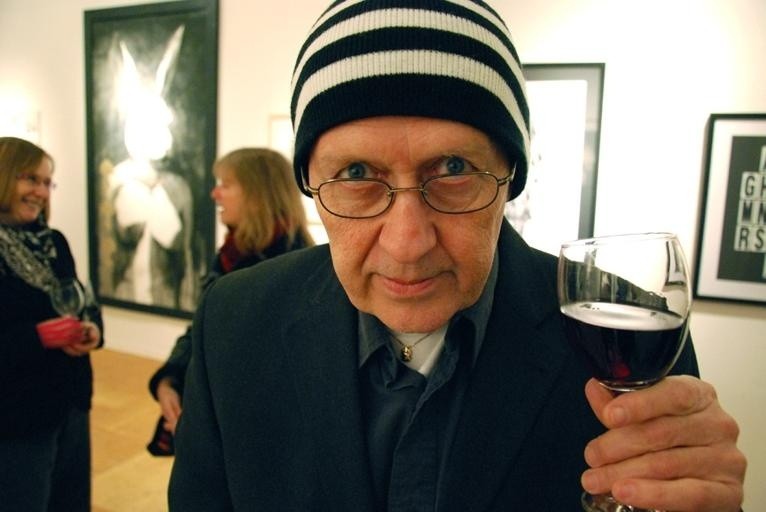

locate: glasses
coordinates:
[299,152,520,223]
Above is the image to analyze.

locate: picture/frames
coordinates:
[84,1,219,317]
[692,114,765,305]
[502,62,605,239]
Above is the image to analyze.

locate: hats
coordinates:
[289,0,533,202]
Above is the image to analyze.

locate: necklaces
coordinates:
[388,332,437,361]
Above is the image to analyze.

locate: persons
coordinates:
[0,136,106,510]
[144,146,315,435]
[166,0,751,512]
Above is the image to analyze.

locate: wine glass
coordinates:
[557,232,693,512]
[47,277,85,319]
